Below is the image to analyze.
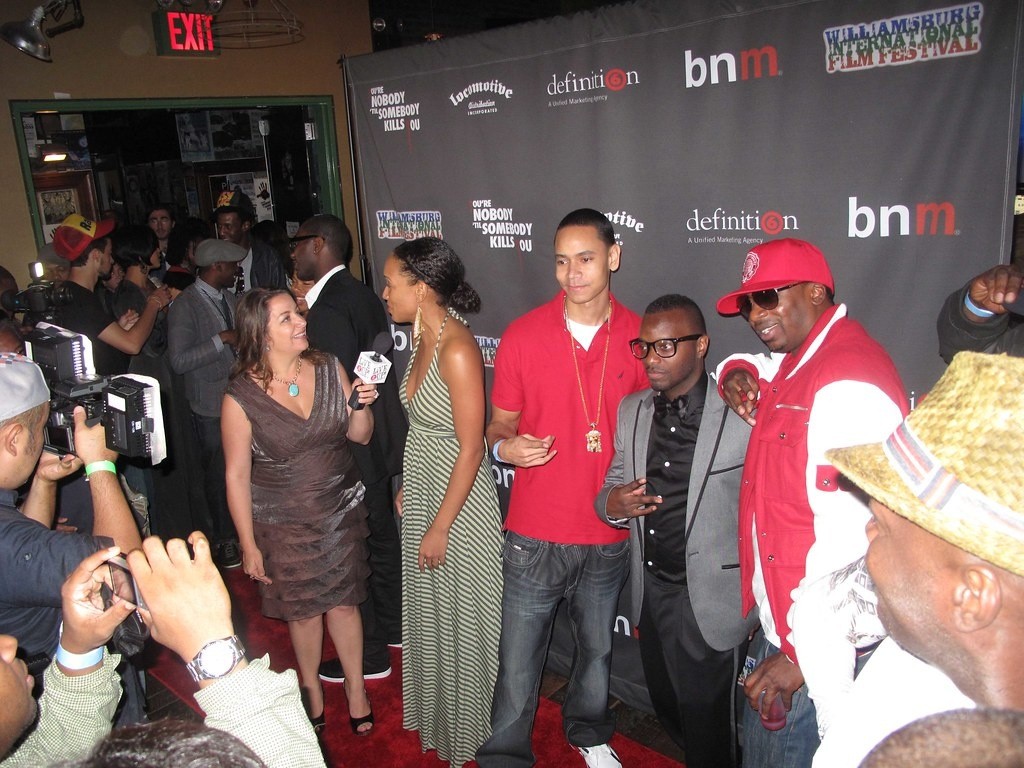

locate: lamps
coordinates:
[372,18,406,50]
[0,0,84,63]
[152,0,223,57]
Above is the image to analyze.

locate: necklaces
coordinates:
[564,295,611,452]
[273,356,302,397]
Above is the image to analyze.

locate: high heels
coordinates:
[305,682,326,732]
[341,674,373,737]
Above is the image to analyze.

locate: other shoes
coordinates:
[213,542,243,568]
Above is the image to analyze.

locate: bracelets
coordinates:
[493,439,506,463]
[147,294,163,311]
[57,641,104,670]
[964,290,994,317]
[85,460,117,477]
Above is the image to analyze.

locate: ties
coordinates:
[220,295,237,360]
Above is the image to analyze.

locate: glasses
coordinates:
[286,235,324,251]
[234,265,245,291]
[629,334,700,358]
[737,282,808,321]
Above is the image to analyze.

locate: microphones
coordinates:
[348,332,393,410]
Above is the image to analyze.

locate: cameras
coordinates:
[108,552,150,612]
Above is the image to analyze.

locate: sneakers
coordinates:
[569,741,623,768]
[387,633,404,648]
[317,656,391,682]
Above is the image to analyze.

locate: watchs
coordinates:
[186,635,246,682]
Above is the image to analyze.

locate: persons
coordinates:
[0,191,1024,768]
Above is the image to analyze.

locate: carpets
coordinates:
[143,564,689,768]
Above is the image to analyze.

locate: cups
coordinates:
[758,689,787,731]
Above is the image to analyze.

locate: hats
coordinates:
[194,239,248,266]
[0,352,50,421]
[717,238,834,315]
[53,214,115,262]
[823,351,1024,574]
